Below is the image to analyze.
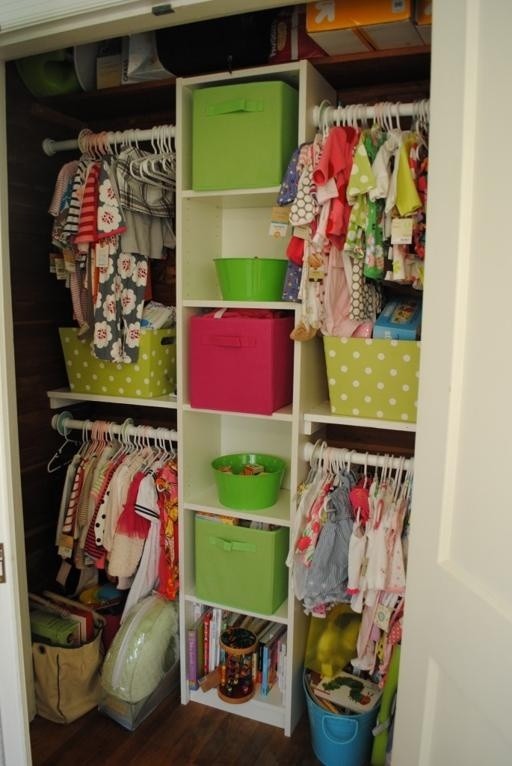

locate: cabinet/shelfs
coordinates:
[170,57,306,740]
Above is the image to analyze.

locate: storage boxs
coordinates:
[304,0,431,57]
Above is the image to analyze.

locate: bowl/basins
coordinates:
[211,257,288,302]
[209,452,289,510]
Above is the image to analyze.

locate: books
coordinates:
[30,586,102,655]
[186,605,287,705]
[303,666,383,719]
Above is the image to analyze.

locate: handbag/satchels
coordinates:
[32,611,107,724]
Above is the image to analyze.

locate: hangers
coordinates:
[297,441,414,534]
[127,125,175,191]
[311,97,427,162]
[46,418,177,491]
